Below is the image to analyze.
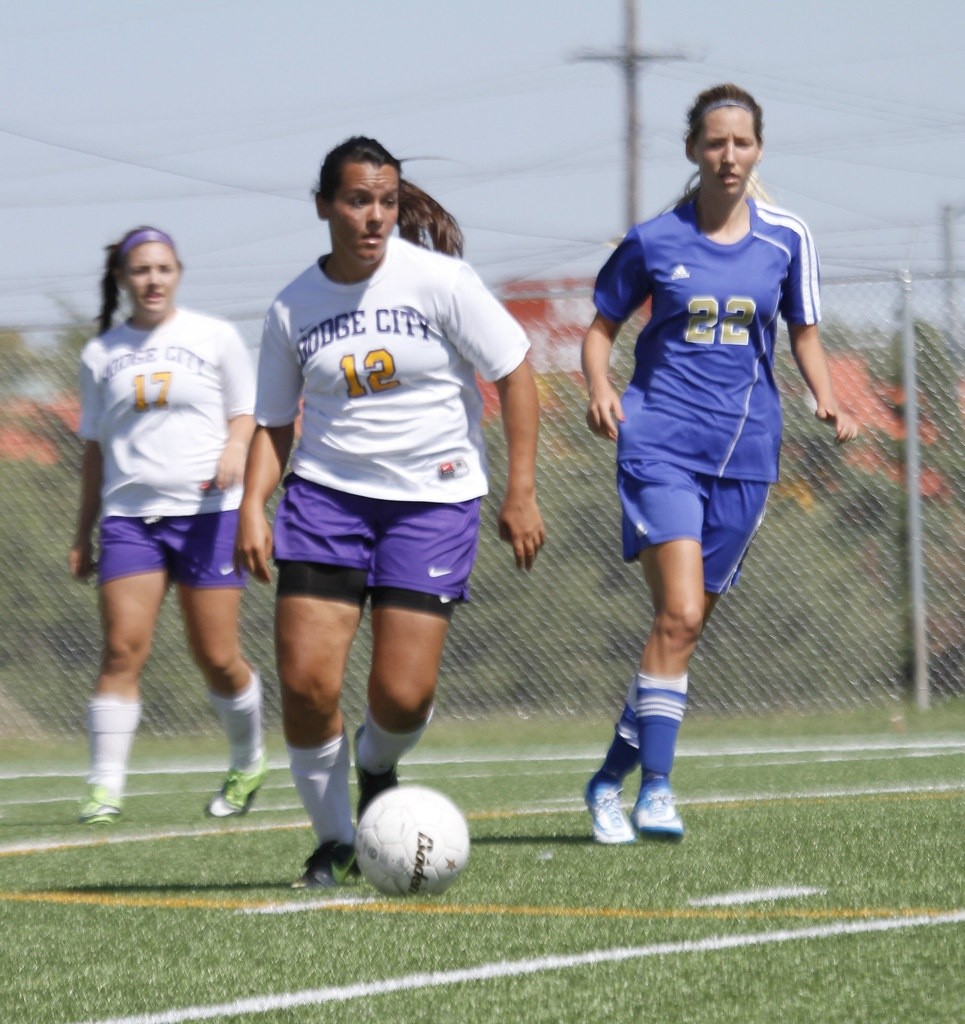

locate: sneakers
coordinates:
[354,725,399,826]
[582,774,685,848]
[289,840,363,890]
[79,798,125,827]
[204,766,273,819]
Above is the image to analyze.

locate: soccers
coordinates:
[358,782,471,902]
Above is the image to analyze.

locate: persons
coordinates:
[69,225,264,826]
[580,84,859,845]
[230,138,547,893]
